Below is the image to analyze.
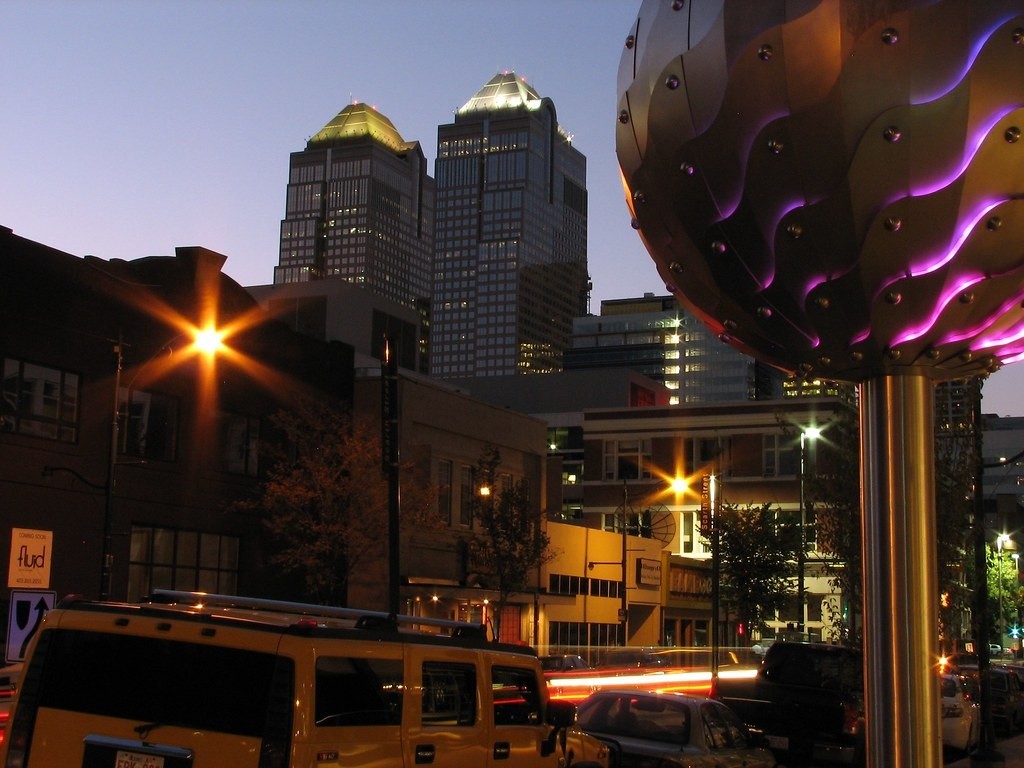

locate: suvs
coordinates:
[1,589,612,768]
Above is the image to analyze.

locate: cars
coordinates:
[575,690,780,768]
[538,655,593,672]
[704,639,1024,756]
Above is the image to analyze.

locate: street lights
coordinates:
[997,534,1009,663]
[39,318,232,601]
[587,469,688,647]
[794,422,821,641]
